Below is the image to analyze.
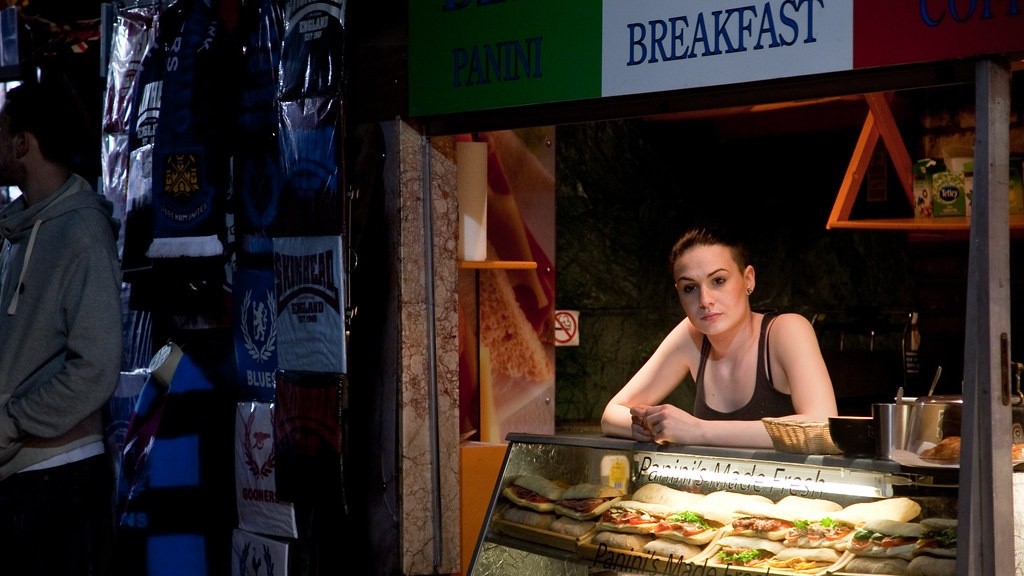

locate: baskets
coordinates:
[762,417,844,454]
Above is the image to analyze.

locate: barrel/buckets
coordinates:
[872,396,963,461]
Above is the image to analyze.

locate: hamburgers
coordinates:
[502,474,963,573]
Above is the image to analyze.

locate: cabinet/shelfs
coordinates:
[462,431,961,576]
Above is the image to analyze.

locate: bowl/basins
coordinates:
[828,416,875,458]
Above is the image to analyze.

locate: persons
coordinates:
[0,81,127,576]
[595,224,845,455]
